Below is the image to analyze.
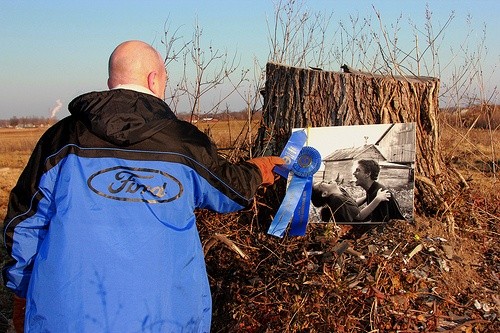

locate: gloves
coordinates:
[13,293,26,333]
[246,156,286,193]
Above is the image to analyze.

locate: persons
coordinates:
[311,179,391,222]
[0,40,285,333]
[353,159,406,222]
